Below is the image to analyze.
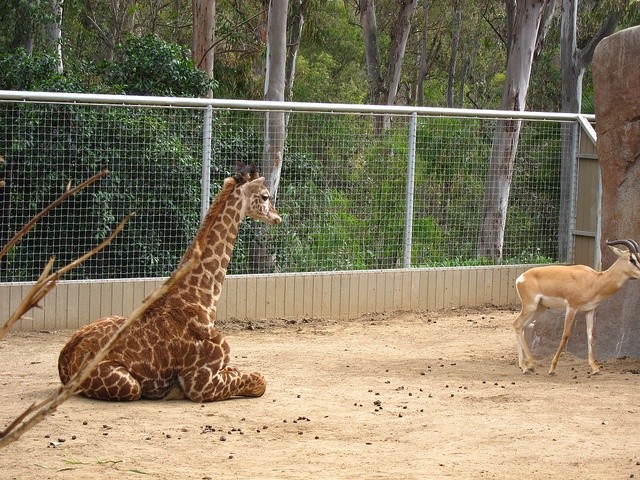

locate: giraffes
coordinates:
[58,162,283,402]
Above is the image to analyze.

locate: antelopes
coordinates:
[511,237,640,377]
